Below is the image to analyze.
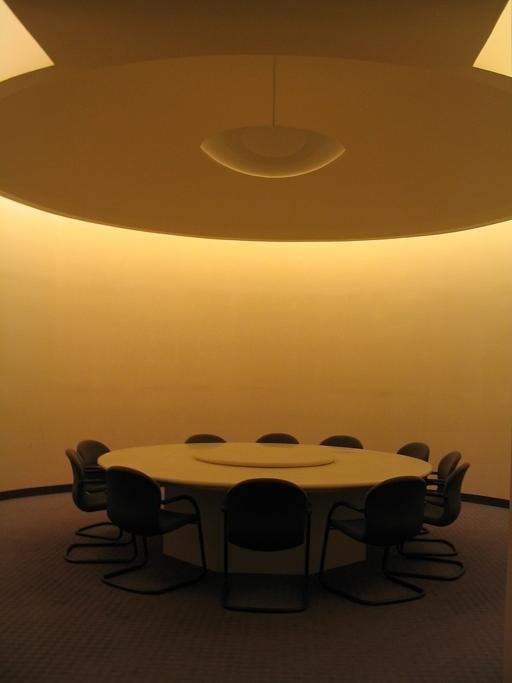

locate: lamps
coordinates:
[201,58,345,181]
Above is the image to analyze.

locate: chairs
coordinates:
[185,433,226,443]
[256,433,300,444]
[423,450,462,483]
[63,449,139,565]
[216,477,312,613]
[380,461,471,580]
[396,462,470,558]
[396,441,431,461]
[317,475,427,606]
[73,440,126,541]
[319,435,364,449]
[100,465,208,596]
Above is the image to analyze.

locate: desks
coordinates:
[96,441,434,577]
